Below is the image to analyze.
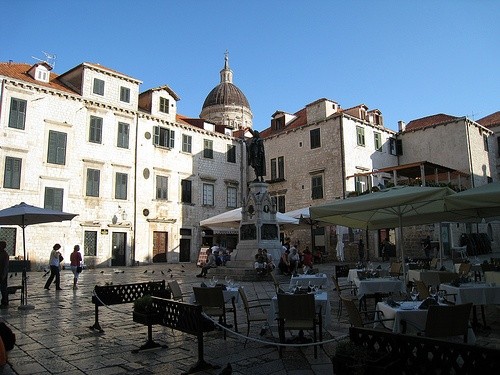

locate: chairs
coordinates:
[168,281,194,302]
[388,262,403,280]
[413,278,457,305]
[193,287,234,340]
[238,286,272,350]
[417,302,473,343]
[274,293,322,359]
[270,268,290,294]
[331,276,360,321]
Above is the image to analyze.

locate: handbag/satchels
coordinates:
[58,265,61,271]
[77,266,82,273]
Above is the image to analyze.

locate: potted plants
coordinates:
[331,340,371,375]
[132,295,161,325]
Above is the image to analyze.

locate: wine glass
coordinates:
[309,281,315,293]
[411,287,419,306]
[292,284,297,293]
[314,284,319,294]
[296,281,302,291]
[303,266,308,276]
[364,267,376,280]
[225,276,231,288]
[417,260,433,271]
[213,276,218,285]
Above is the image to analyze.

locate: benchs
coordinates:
[333,264,353,286]
[405,270,458,290]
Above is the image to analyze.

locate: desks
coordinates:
[385,263,418,273]
[352,278,406,310]
[347,268,385,293]
[440,281,500,336]
[188,287,243,340]
[265,289,332,347]
[373,302,476,346]
[289,273,328,288]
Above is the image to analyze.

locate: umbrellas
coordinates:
[336,225,344,265]
[443,181,500,223]
[283,204,322,256]
[308,184,457,292]
[199,207,300,233]
[42,270,50,278]
[0,202,79,305]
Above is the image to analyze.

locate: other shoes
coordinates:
[197,275,202,277]
[44,287,50,290]
[56,287,62,290]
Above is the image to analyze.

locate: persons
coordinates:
[381,238,389,262]
[254,248,275,275]
[0,241,9,310]
[423,235,433,260]
[279,237,314,276]
[44,244,62,291]
[196,244,221,277]
[70,245,82,290]
[460,233,472,262]
[358,238,364,264]
[247,127,266,182]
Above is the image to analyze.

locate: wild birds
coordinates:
[99,270,124,274]
[10,272,18,279]
[143,264,184,278]
